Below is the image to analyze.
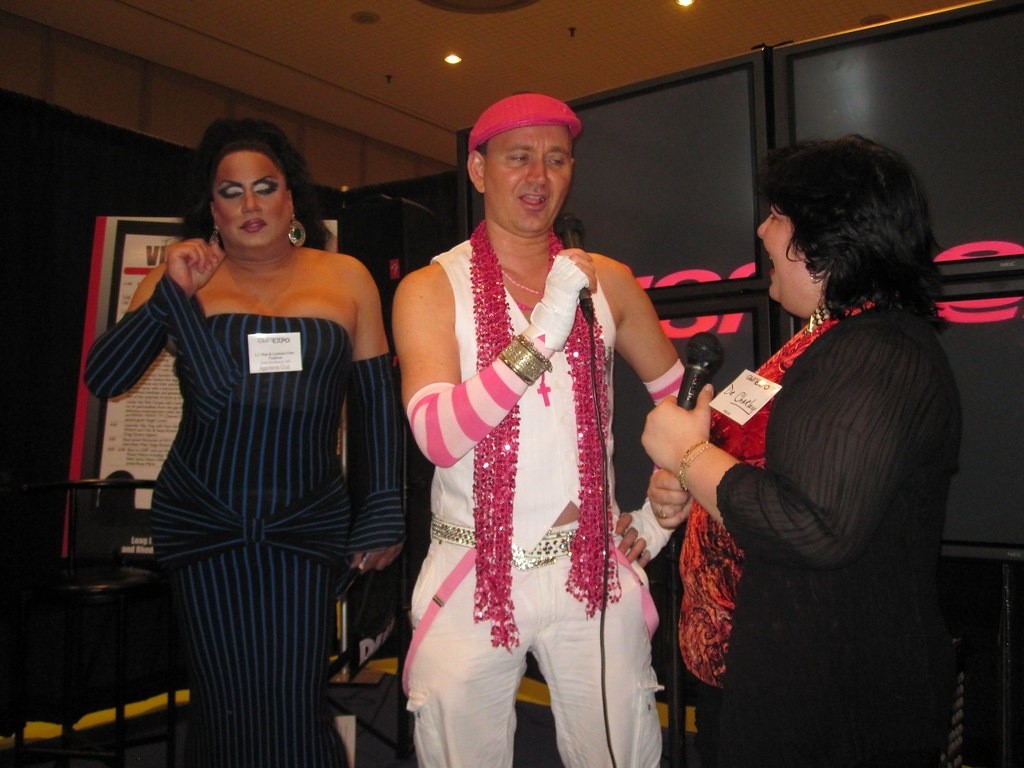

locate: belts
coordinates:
[431,515,577,569]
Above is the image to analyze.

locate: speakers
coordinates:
[337,197,444,377]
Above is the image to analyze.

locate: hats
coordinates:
[468,92,584,153]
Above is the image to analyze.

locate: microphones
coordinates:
[553,211,594,323]
[677,333,725,411]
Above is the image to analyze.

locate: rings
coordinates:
[657,506,666,518]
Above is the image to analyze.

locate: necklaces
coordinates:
[501,270,541,310]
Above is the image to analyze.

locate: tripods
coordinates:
[319,422,414,757]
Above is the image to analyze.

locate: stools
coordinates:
[0,478,177,768]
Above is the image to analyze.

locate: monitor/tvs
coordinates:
[455,0,1024,563]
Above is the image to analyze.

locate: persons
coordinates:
[642,135,962,768]
[84,118,404,768]
[392,95,685,768]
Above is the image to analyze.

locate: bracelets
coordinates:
[679,441,713,490]
[498,335,551,384]
